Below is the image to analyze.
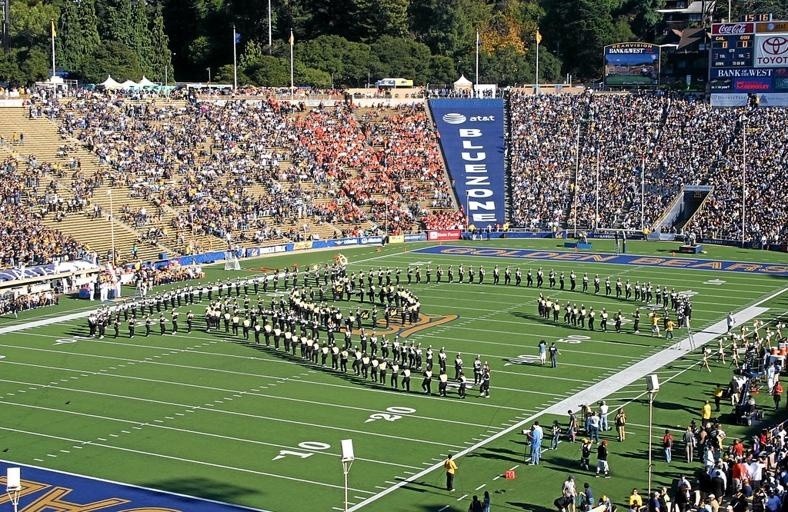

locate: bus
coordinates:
[375,77,412,93]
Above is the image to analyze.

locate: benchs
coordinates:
[1,104,456,270]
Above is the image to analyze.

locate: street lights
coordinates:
[4,467,25,512]
[165,52,178,85]
[643,371,660,498]
[739,112,749,248]
[106,187,116,266]
[338,437,355,512]
[205,67,211,81]
